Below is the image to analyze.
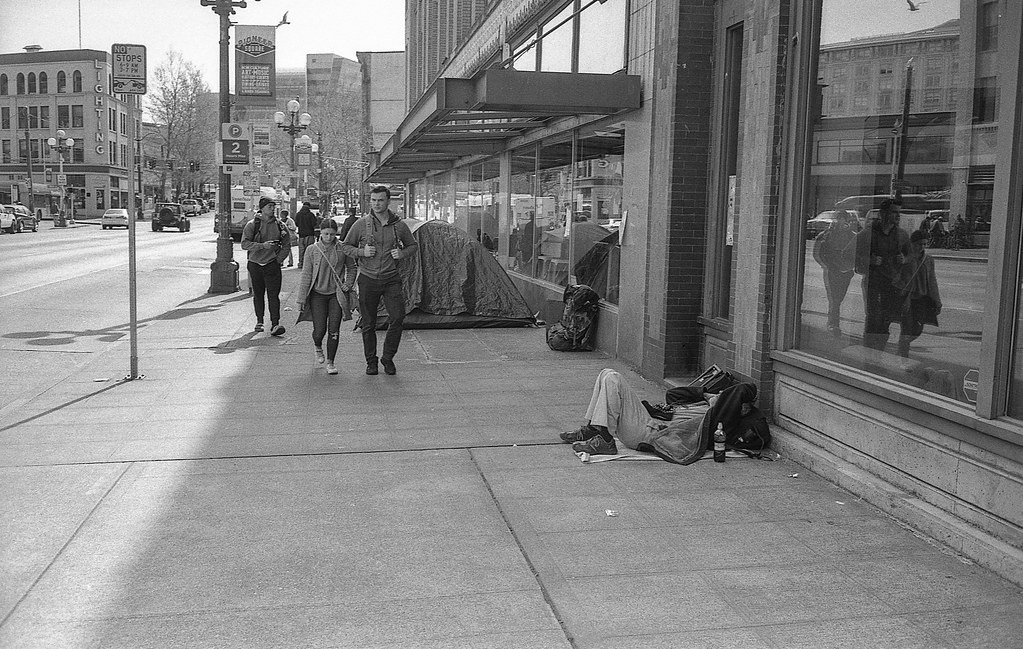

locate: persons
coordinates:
[241,197,291,335]
[278,209,296,266]
[920,216,930,231]
[296,201,318,268]
[296,219,353,374]
[560,368,758,466]
[342,186,418,375]
[314,213,322,242]
[855,199,917,352]
[892,230,942,358]
[340,208,358,242]
[954,214,964,234]
[934,216,944,230]
[51,201,60,225]
[813,209,857,335]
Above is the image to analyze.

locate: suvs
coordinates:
[151,203,190,233]
[806,211,862,238]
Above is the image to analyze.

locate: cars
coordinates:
[924,209,950,232]
[180,198,215,216]
[101,208,129,229]
[0,204,17,234]
[3,205,39,232]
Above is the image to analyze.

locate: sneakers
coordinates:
[560,424,603,443]
[571,435,618,456]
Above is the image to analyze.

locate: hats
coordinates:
[258,196,275,210]
[281,210,289,216]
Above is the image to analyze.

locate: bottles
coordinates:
[714,423,726,462]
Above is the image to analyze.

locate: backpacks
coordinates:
[547,284,599,352]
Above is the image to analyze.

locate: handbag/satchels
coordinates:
[349,291,359,311]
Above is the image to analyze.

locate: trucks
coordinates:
[214,185,283,243]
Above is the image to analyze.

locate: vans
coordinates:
[832,193,930,218]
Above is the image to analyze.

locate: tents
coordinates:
[353,218,537,331]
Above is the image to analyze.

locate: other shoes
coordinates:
[366,362,378,375]
[255,323,264,332]
[315,345,325,363]
[381,358,395,375]
[271,325,285,336]
[288,264,293,267]
[327,363,338,374]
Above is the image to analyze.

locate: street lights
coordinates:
[47,130,74,227]
[273,100,312,221]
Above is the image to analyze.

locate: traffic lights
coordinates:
[190,160,195,172]
[168,161,174,171]
[152,159,157,168]
[195,160,200,171]
[148,159,153,169]
